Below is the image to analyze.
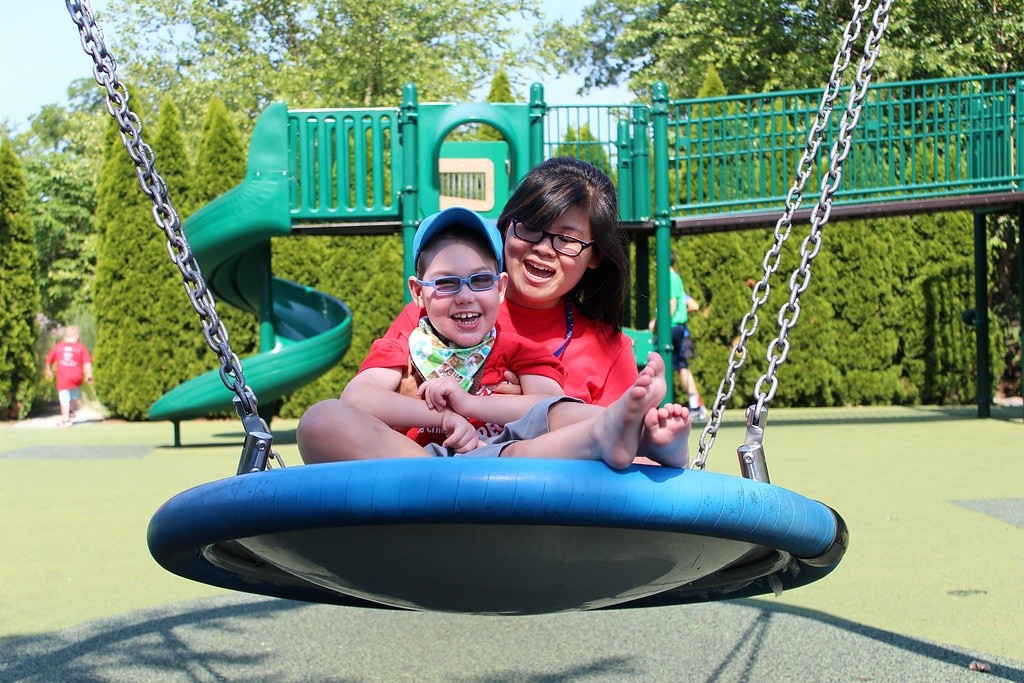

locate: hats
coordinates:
[411,205,504,273]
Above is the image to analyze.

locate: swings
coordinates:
[66,0,892,619]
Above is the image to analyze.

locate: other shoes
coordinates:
[57,420,71,428]
[71,415,75,420]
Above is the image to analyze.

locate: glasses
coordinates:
[511,217,596,258]
[416,272,501,296]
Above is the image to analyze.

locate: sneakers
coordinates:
[689,406,708,422]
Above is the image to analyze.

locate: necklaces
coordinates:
[550,301,573,362]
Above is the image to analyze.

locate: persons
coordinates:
[339,206,692,469]
[649,250,707,421]
[297,157,639,465]
[45,325,93,428]
